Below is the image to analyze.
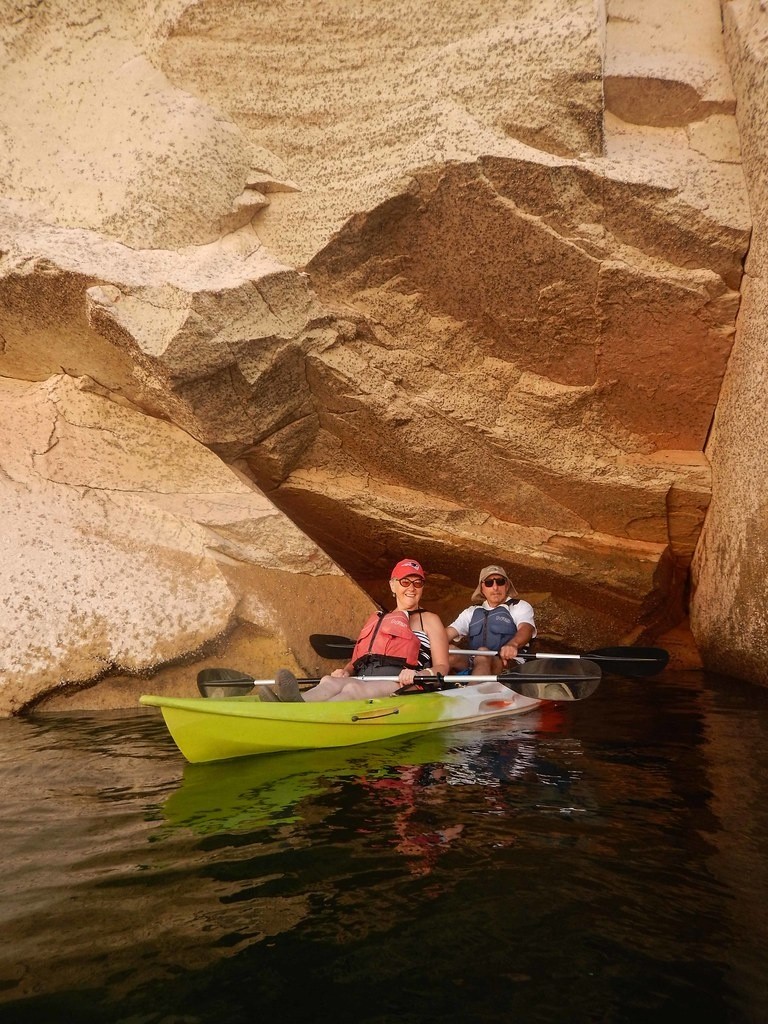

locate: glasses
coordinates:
[395,578,425,588]
[481,578,506,587]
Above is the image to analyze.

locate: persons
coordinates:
[445,564,538,689]
[260,558,451,703]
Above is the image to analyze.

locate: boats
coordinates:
[138,694,543,765]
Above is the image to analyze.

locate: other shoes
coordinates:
[275,669,304,702]
[259,685,279,702]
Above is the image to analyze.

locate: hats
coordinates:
[471,565,519,602]
[391,559,425,580]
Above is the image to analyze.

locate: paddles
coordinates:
[309,633,671,675]
[196,658,602,701]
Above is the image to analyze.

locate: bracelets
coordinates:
[425,668,434,684]
